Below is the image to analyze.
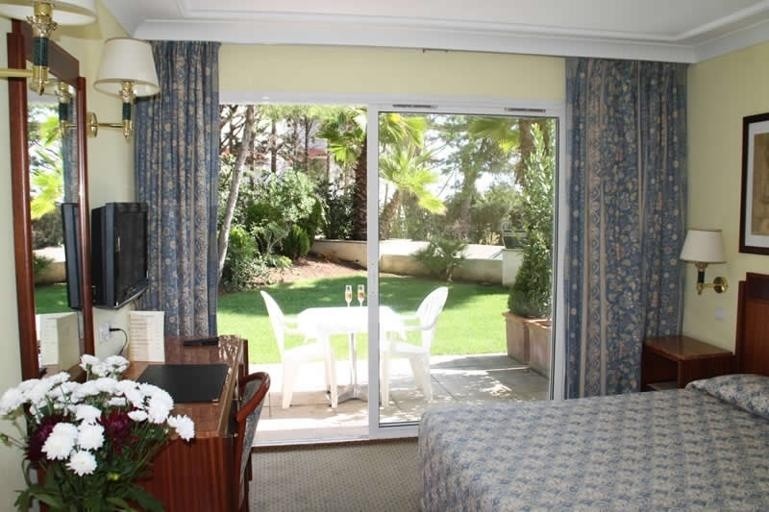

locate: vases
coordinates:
[526,318,555,379]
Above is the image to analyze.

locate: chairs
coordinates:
[234,373,271,511]
[381,287,448,408]
[261,290,335,410]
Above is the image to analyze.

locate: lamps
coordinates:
[86,38,162,137]
[676,229,728,295]
[1,0,99,96]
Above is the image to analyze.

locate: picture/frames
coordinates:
[739,112,768,255]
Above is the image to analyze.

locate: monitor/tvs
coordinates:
[61,203,83,310]
[90,202,149,310]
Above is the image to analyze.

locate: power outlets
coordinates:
[105,321,112,341]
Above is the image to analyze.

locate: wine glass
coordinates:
[345,285,353,315]
[357,284,365,316]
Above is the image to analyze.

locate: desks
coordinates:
[90,335,247,511]
[301,303,403,408]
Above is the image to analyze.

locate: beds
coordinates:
[419,370,768,511]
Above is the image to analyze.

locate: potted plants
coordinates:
[500,121,554,363]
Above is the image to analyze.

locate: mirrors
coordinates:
[26,62,77,139]
[6,16,96,386]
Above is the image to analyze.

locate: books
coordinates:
[36,311,80,371]
[125,312,168,364]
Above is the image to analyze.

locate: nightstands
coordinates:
[640,333,734,390]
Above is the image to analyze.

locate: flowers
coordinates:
[0,356,197,511]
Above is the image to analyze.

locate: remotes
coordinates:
[183,337,219,346]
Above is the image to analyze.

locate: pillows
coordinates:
[689,374,769,415]
